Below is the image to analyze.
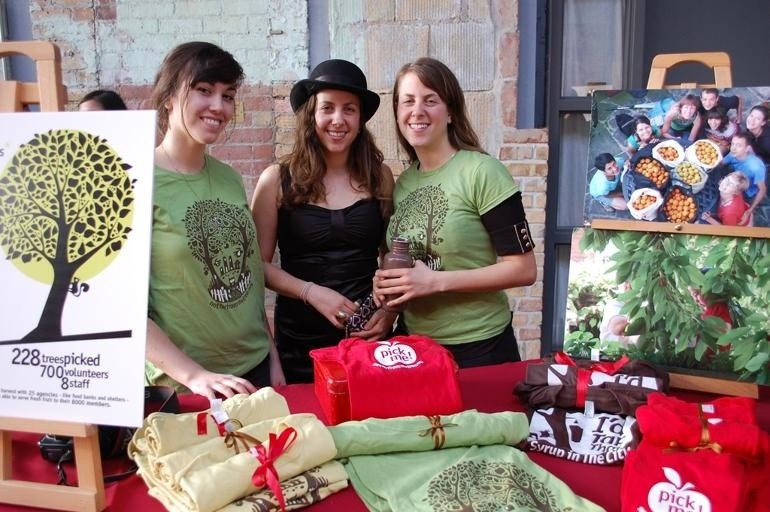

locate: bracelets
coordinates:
[297,280,317,302]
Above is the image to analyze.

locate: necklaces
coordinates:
[161,142,219,227]
[415,148,457,202]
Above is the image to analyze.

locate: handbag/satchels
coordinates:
[37,385,180,493]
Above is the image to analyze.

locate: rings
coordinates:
[336,310,352,322]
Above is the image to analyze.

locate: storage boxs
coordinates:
[312,353,460,428]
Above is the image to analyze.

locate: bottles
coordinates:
[378,237,416,312]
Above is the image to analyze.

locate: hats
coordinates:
[289,58,382,126]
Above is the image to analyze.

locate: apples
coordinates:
[696,142,718,166]
[658,148,679,161]
[634,158,670,189]
[676,163,702,185]
[662,190,696,223]
[633,195,657,210]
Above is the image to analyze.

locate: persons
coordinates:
[138,40,291,409]
[368,56,541,368]
[253,61,401,386]
[685,267,736,365]
[578,284,605,336]
[586,88,770,227]
[76,89,131,112]
[600,274,656,354]
